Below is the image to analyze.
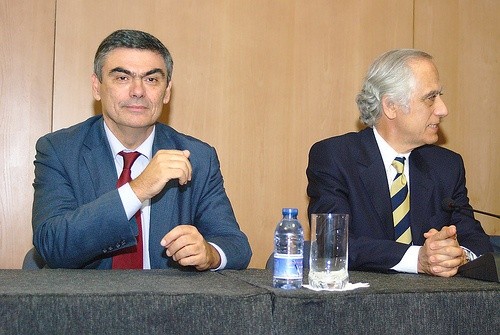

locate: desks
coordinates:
[0,269,500,335]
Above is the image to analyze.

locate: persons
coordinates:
[31,29,253,271]
[306,49,499,282]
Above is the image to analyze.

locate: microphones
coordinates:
[442,198,500,219]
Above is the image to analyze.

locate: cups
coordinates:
[310,214,350,288]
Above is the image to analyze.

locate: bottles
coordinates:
[272,208,304,290]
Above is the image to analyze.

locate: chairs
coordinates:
[267,241,310,270]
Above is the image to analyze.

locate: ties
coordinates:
[391,157,412,244]
[113,151,142,270]
